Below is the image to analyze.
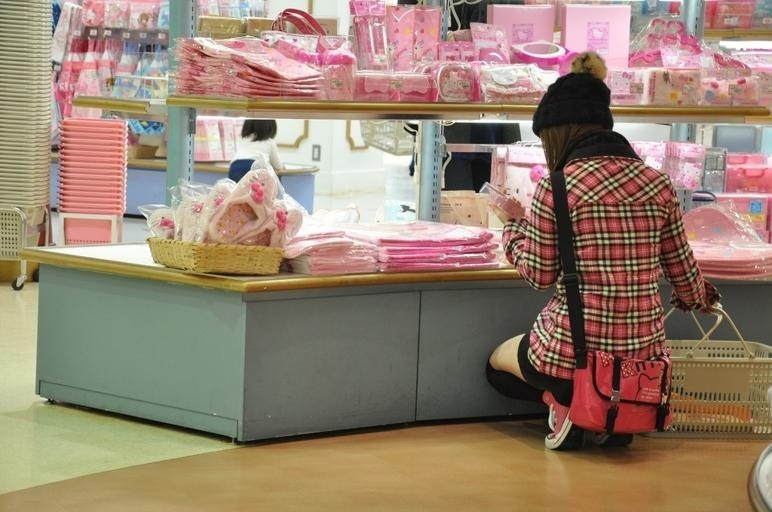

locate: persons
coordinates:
[483,52,724,452]
[228,118,281,182]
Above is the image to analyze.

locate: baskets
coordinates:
[145,236,287,277]
[664,300,772,439]
[1,0,127,261]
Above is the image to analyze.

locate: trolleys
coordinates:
[2,206,53,291]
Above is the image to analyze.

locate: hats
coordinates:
[531,70,615,136]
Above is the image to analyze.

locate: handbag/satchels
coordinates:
[262,8,354,99]
[549,164,674,436]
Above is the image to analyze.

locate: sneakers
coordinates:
[541,391,610,449]
